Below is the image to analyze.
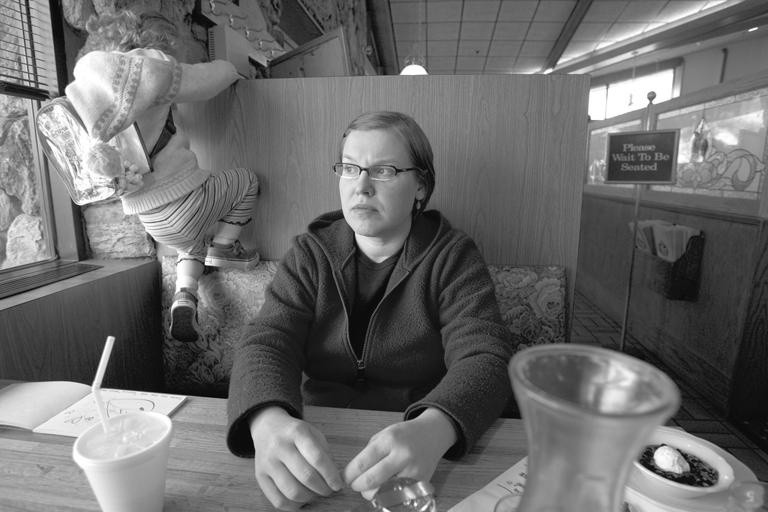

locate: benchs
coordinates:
[158,252,577,420]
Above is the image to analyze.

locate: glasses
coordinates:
[332,163,425,182]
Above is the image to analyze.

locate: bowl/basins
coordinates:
[635,432,733,500]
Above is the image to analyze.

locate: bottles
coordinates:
[509,339,680,512]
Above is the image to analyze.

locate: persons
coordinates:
[66,9,263,344]
[225,110,517,512]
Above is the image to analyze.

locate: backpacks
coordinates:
[36,97,176,207]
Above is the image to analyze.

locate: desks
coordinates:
[0,375,688,512]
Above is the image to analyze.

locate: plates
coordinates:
[602,423,763,512]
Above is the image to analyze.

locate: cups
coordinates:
[72,411,169,512]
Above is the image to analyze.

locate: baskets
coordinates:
[635,223,704,302]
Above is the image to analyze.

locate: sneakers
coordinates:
[205,240,260,269]
[169,288,201,341]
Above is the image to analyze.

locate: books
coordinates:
[0,381,189,446]
